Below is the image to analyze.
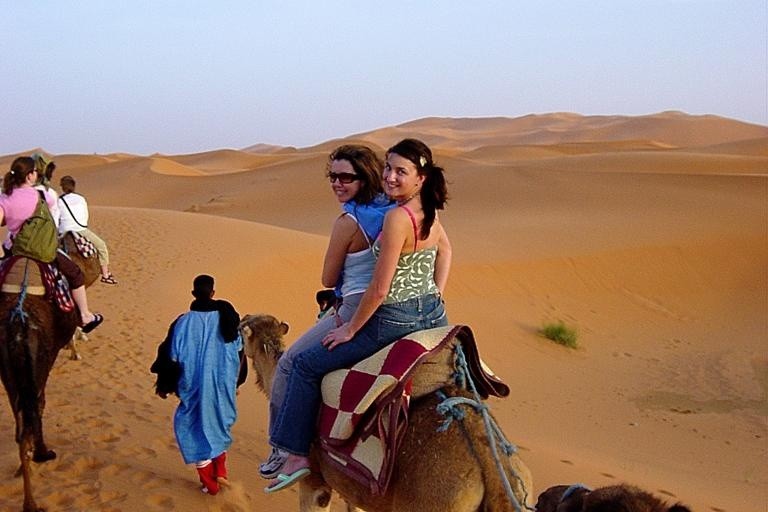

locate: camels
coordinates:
[533,480,692,512]
[0,248,81,512]
[58,230,103,361]
[237,311,535,512]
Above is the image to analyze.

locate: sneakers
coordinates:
[201,485,208,494]
[215,476,230,486]
[257,446,311,494]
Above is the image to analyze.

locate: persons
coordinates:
[266,138,451,493]
[258,145,398,479]
[58,176,118,284]
[151,275,248,495]
[0,153,103,334]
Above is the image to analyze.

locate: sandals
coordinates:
[81,313,103,334]
[100,273,118,284]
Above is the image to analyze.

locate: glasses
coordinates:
[330,172,359,183]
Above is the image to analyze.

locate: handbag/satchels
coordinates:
[11,199,58,264]
